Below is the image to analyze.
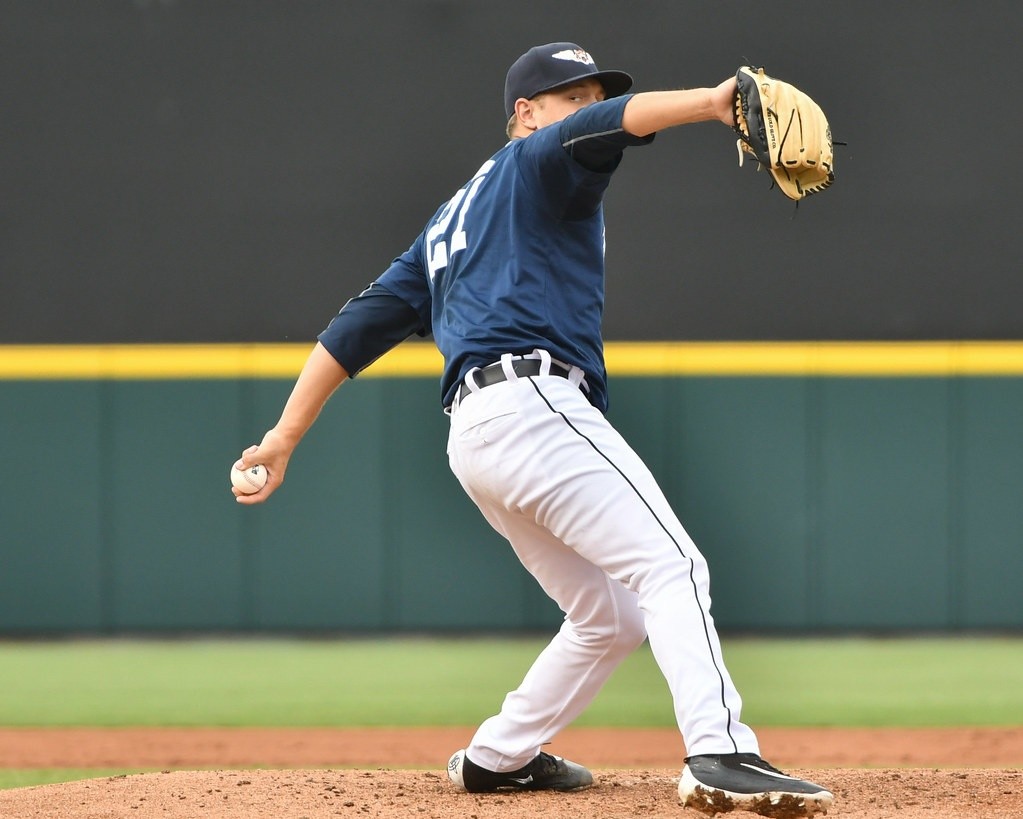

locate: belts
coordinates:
[459,359,595,408]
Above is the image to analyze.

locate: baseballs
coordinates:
[229,457,268,495]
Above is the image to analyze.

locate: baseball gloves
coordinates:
[728,54,850,223]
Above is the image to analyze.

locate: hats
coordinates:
[504,43,633,120]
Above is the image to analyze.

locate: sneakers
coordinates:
[448,749,594,792]
[678,755,833,807]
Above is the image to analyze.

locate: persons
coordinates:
[230,44,834,810]
